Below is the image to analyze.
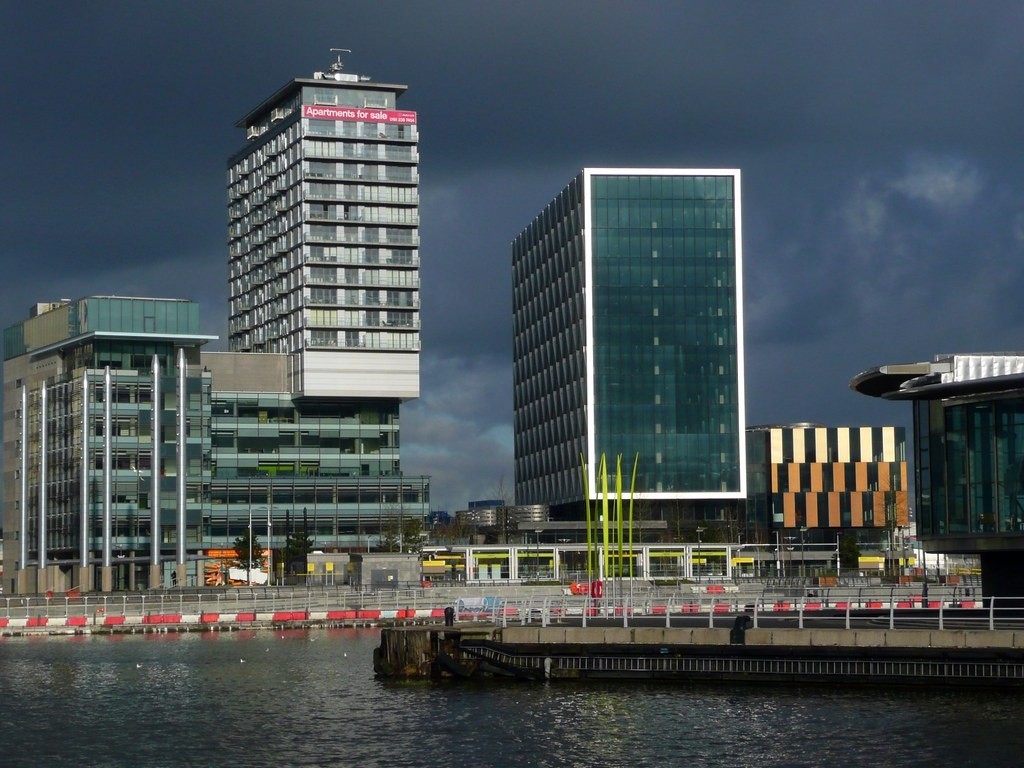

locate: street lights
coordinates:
[801,528,808,577]
[535,530,542,581]
[737,533,743,578]
[837,531,843,576]
[775,548,780,577]
[267,523,272,586]
[368,535,371,553]
[696,528,703,584]
[248,524,253,587]
[784,537,798,577]
[558,539,571,585]
